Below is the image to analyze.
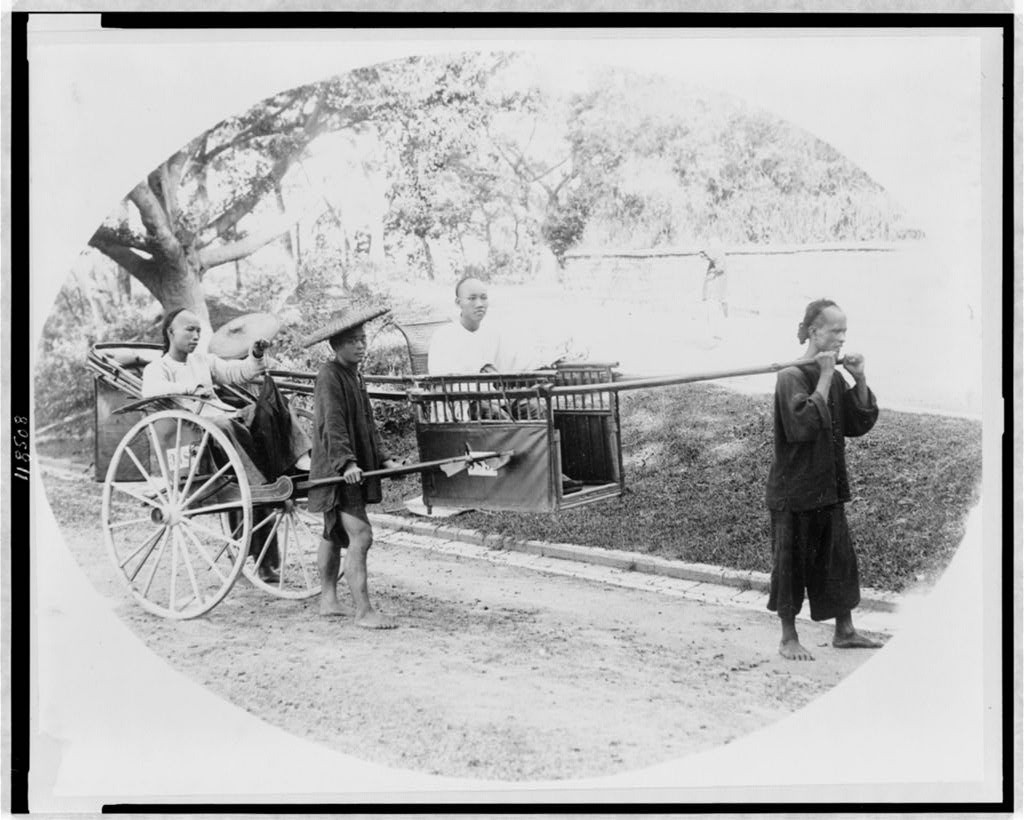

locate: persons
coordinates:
[308,324,406,630]
[765,298,884,661]
[427,277,584,496]
[141,308,310,483]
[208,311,290,584]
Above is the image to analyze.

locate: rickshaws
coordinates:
[85,341,505,623]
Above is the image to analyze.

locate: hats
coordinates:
[209,313,285,360]
[302,307,390,348]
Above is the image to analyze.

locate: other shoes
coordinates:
[562,472,584,492]
